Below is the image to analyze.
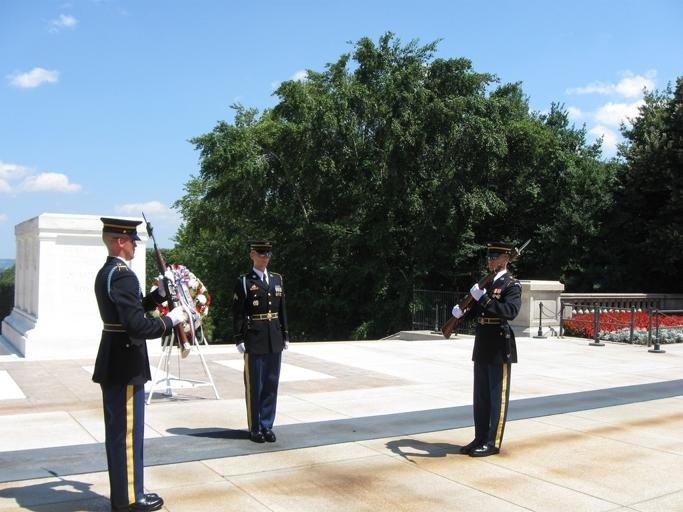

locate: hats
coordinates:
[100,217,143,241]
[484,242,513,257]
[251,240,273,257]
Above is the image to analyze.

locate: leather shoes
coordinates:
[263,430,276,442]
[460,441,480,454]
[469,443,499,457]
[111,498,163,511]
[250,431,265,443]
[145,493,158,498]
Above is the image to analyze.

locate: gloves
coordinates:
[470,283,486,301]
[236,342,246,353]
[452,304,464,318]
[157,271,175,298]
[283,341,289,350]
[166,306,186,327]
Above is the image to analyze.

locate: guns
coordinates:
[142,212,190,359]
[442,238,532,339]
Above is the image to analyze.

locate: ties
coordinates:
[263,273,267,285]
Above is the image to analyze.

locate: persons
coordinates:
[234,241,288,443]
[451,242,522,457]
[92,216,187,512]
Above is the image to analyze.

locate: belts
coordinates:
[477,317,507,324]
[252,312,278,320]
[103,323,127,332]
